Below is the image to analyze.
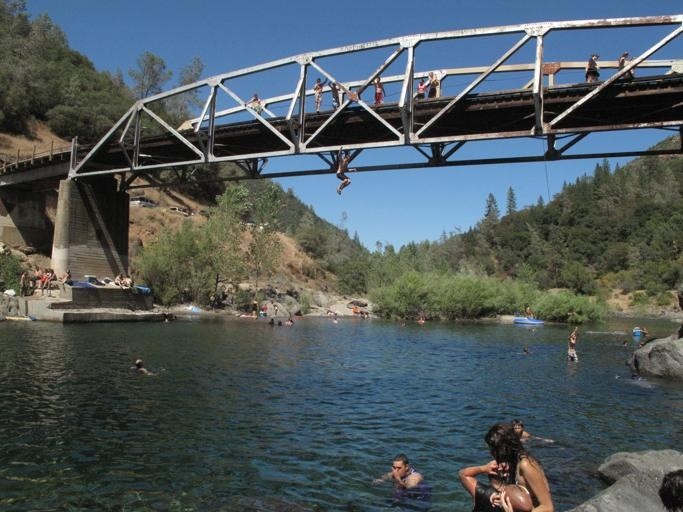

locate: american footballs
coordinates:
[504,484,533,511]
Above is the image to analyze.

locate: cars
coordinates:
[244,220,271,229]
[169,206,189,217]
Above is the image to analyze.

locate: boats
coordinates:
[511,317,544,325]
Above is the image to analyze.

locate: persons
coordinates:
[165,313,177,323]
[114,273,126,288]
[458,459,533,512]
[640,327,650,337]
[98,277,114,285]
[254,299,426,328]
[622,340,628,347]
[17,264,72,297]
[335,147,356,195]
[523,347,529,355]
[482,421,554,511]
[122,274,133,287]
[416,78,427,101]
[524,306,533,320]
[510,418,554,447]
[585,54,600,83]
[130,358,158,377]
[626,56,636,79]
[617,51,628,80]
[328,81,341,110]
[312,77,327,112]
[427,71,439,99]
[372,77,386,105]
[566,326,579,363]
[370,452,431,511]
[657,467,682,511]
[244,93,262,120]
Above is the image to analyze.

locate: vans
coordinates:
[130,196,155,209]
